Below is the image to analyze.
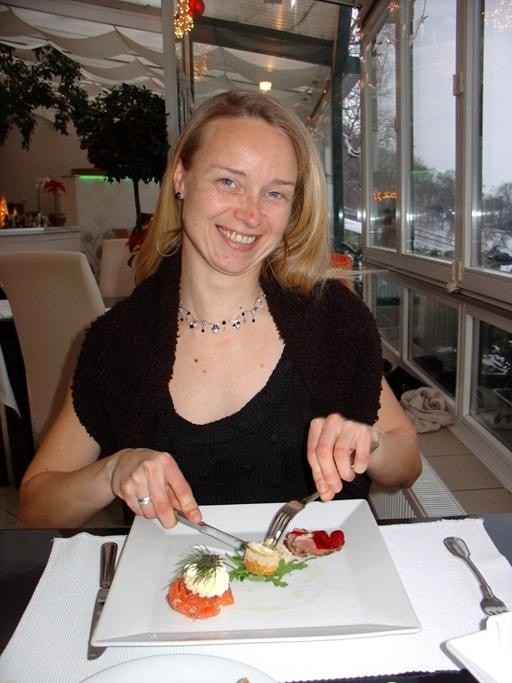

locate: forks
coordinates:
[444,537,510,616]
[263,440,379,549]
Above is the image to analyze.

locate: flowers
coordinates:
[44,178,66,214]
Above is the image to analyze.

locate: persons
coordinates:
[15,88,423,529]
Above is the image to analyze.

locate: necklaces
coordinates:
[179,289,270,335]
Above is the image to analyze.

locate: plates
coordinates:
[79,653,280,683]
[91,499,420,648]
[445,612,510,683]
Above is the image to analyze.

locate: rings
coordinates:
[136,496,155,508]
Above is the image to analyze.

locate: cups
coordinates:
[6,201,25,227]
[23,201,48,228]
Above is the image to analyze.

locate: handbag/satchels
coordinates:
[386,366,422,400]
[399,387,454,434]
[413,355,443,372]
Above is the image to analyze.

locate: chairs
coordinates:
[99,239,138,306]
[0,250,106,456]
[0,346,21,491]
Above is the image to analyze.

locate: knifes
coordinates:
[173,508,247,551]
[87,542,116,660]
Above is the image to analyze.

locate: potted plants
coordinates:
[492,337,512,419]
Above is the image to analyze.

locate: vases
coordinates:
[48,215,66,226]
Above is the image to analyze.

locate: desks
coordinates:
[1,227,84,252]
